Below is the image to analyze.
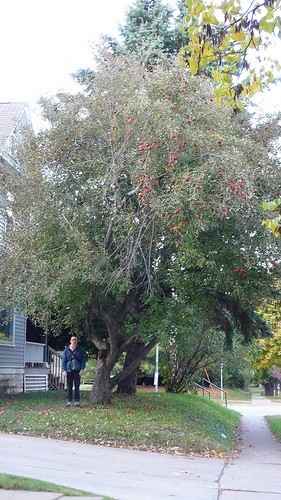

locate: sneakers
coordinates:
[74,402,80,406]
[67,402,71,406]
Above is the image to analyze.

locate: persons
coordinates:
[61,336,86,408]
